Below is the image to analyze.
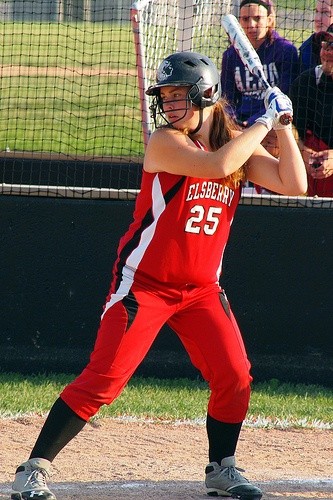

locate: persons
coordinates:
[289,23,333,179]
[297,0,333,68]
[11,53,307,500]
[220,0,299,128]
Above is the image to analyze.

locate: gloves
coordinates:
[254,86,294,130]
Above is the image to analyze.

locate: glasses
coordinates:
[321,41,333,50]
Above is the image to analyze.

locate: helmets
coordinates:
[145,51,222,108]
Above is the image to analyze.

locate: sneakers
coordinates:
[11,456,57,500]
[203,455,263,500]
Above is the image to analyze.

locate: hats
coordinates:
[314,23,333,41]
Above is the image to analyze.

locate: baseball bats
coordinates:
[219,10,294,127]
